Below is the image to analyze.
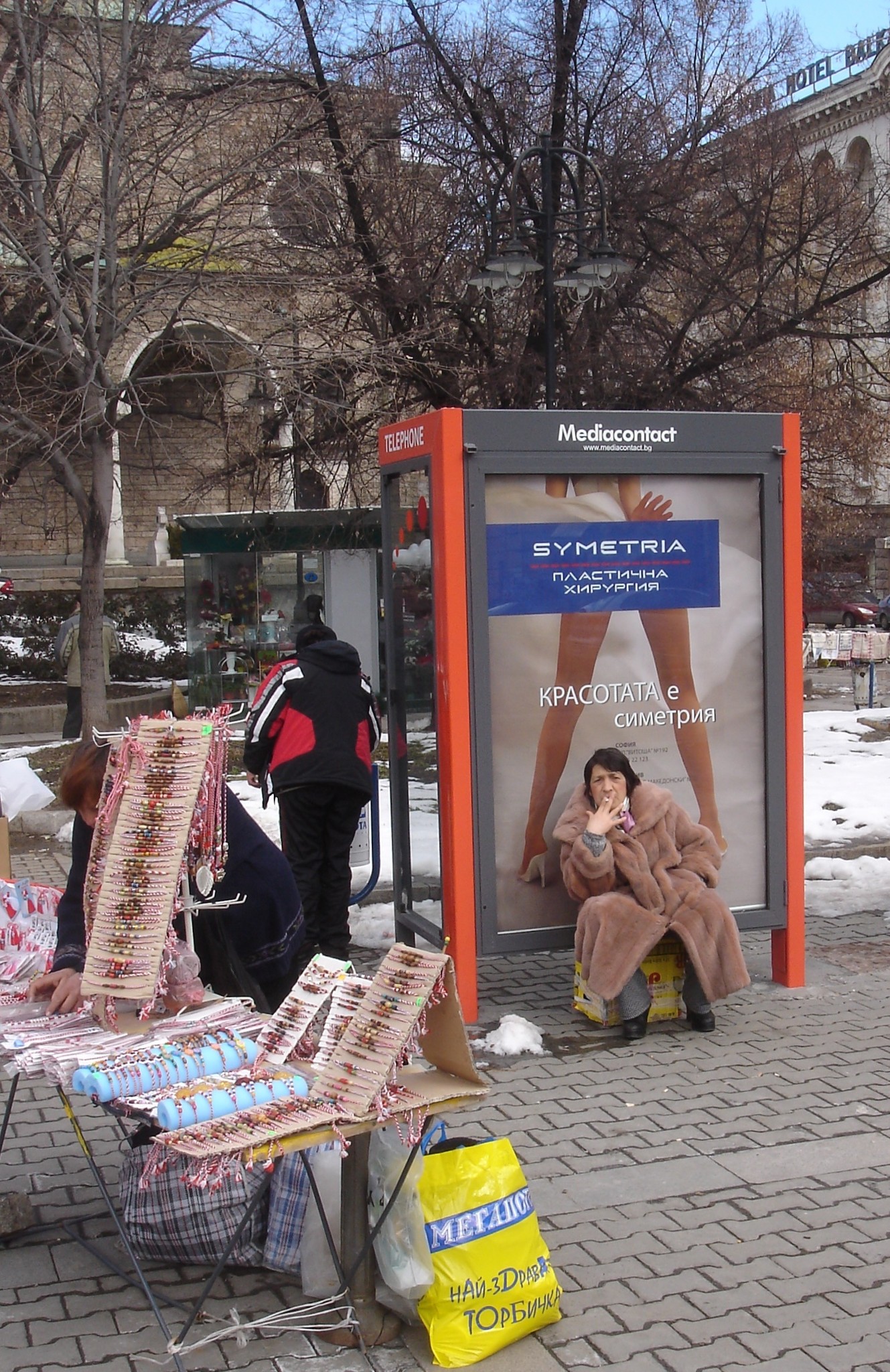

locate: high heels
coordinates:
[621,1000,651,1039]
[684,998,716,1032]
[516,851,548,888]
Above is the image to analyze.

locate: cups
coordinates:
[225,652,236,672]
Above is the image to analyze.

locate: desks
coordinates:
[1,1051,488,1347]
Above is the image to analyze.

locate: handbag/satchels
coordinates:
[367,1124,434,1297]
[120,1130,413,1316]
[396,1121,563,1368]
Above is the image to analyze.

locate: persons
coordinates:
[293,594,326,625]
[552,748,751,1039]
[54,597,119,740]
[515,474,733,890]
[27,740,309,1016]
[243,626,382,963]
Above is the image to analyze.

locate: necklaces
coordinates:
[0,719,448,1188]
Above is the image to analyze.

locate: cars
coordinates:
[803,572,881,629]
[878,595,890,631]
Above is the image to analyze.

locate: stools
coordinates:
[571,935,686,1025]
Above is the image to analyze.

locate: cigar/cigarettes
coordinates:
[604,797,611,802]
